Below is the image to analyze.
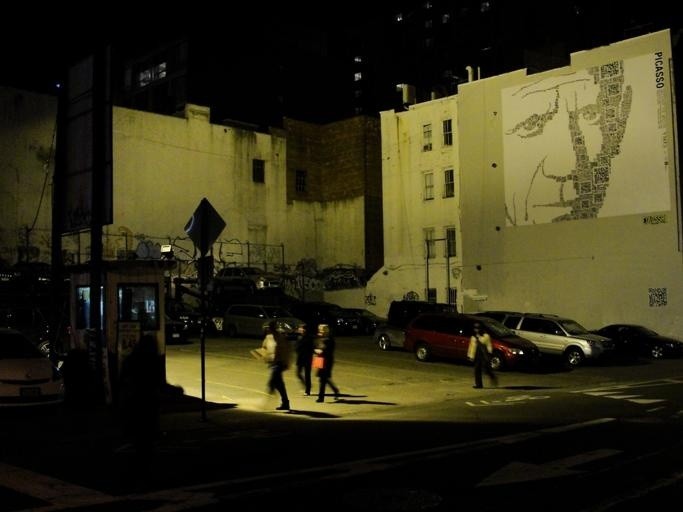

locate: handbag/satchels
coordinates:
[481,345,492,361]
[312,357,323,368]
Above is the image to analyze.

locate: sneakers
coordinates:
[317,399,324,402]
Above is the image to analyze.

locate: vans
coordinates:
[500,312,611,366]
[406,314,539,371]
[387,299,451,328]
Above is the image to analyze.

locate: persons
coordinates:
[466,322,497,389]
[314,321,341,402]
[261,316,289,412]
[500,70,633,225]
[292,322,312,395]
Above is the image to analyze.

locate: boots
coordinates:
[276,400,289,410]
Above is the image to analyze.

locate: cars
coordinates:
[165,267,377,338]
[589,326,680,360]
[377,328,404,351]
[1,329,66,408]
